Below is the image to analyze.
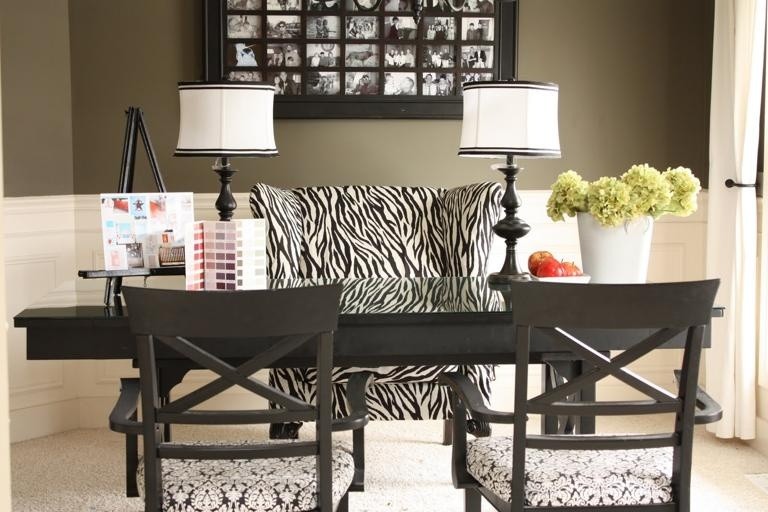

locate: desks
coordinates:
[13,310,733,492]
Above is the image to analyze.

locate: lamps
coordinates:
[174,83,279,217]
[453,78,564,280]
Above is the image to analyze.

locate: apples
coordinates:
[528,250,553,276]
[537,256,567,277]
[561,262,582,276]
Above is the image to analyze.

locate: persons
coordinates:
[227,0,494,96]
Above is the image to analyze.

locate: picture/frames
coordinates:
[200,0,519,120]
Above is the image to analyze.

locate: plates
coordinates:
[528,273,590,284]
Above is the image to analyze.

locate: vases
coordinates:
[575,210,655,285]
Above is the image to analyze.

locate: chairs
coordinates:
[462,275,721,508]
[251,180,501,436]
[111,285,370,510]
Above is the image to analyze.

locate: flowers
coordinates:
[542,165,704,229]
